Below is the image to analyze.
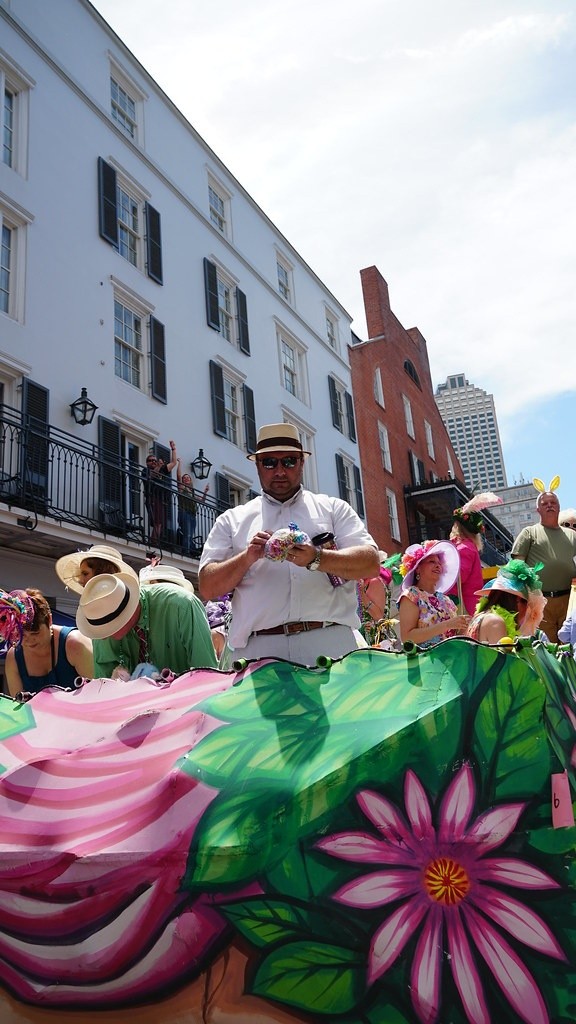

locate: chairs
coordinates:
[0,471,51,516]
[175,527,205,558]
[100,500,145,543]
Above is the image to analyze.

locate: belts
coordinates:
[543,589,571,598]
[250,620,341,637]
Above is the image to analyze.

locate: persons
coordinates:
[511,476,576,664]
[198,423,380,671]
[141,440,176,545]
[177,457,209,557]
[0,545,234,699]
[357,492,549,653]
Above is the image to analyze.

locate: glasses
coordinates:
[512,595,527,604]
[147,458,157,462]
[563,522,576,529]
[258,455,302,470]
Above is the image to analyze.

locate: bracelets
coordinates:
[171,448,176,450]
[364,601,373,612]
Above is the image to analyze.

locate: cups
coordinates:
[311,532,350,588]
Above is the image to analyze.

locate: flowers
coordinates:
[454,508,487,532]
[399,539,446,578]
[499,561,548,593]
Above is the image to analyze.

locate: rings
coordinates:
[293,556,295,561]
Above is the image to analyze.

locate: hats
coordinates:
[141,565,195,594]
[76,572,140,639]
[453,491,503,535]
[402,539,461,590]
[246,423,312,463]
[474,559,543,602]
[55,545,140,595]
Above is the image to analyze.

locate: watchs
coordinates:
[306,547,320,572]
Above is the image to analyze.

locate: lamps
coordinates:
[191,448,212,479]
[70,388,98,425]
